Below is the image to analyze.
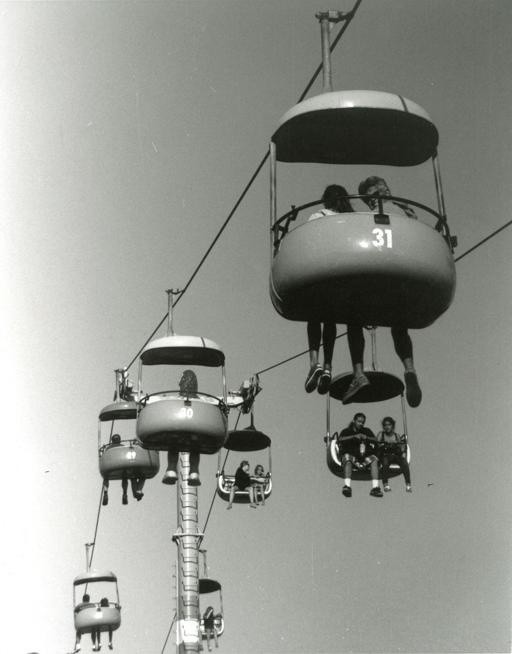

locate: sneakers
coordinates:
[162,470,179,485]
[187,471,202,487]
[403,369,423,408]
[304,362,332,395]
[75,643,113,653]
[122,491,144,505]
[342,485,352,497]
[102,494,109,505]
[405,485,412,493]
[384,485,392,492]
[342,375,371,405]
[369,487,384,497]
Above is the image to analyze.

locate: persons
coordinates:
[377,415,413,493]
[96,597,115,651]
[337,412,385,498]
[342,172,423,409]
[253,463,269,507]
[225,459,258,514]
[303,183,359,394]
[130,435,146,503]
[70,593,98,654]
[203,605,220,652]
[161,368,203,486]
[99,432,130,505]
